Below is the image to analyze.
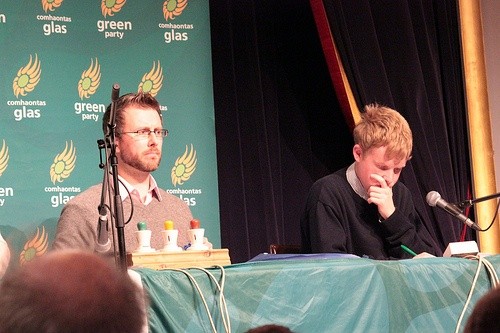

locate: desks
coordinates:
[126,253,500,333]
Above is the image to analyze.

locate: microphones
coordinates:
[425,191,482,231]
[94,204,111,254]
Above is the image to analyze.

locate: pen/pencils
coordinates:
[401,244,417,256]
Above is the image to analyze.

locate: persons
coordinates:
[245,325,292,333]
[299,103,444,258]
[51,90,213,257]
[0,234,145,333]
[463,286,500,333]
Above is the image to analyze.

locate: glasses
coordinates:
[122,128,168,138]
